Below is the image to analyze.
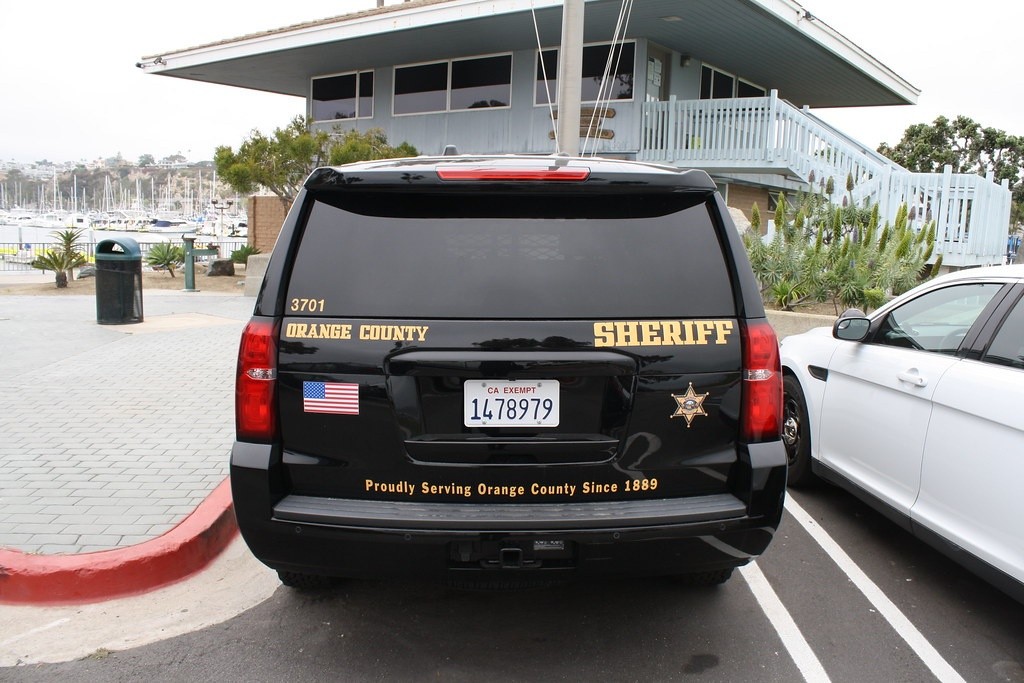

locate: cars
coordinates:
[775,262,1024,617]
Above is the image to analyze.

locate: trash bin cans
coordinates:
[94,238,145,327]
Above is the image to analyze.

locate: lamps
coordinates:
[136,63,145,67]
[153,57,167,66]
[796,10,815,24]
[680,54,689,67]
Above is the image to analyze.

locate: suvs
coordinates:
[228,142,789,596]
